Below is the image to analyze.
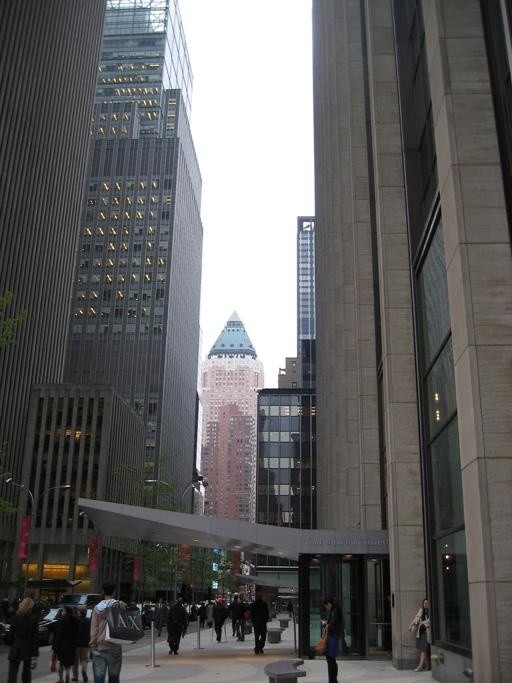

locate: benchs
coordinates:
[264,659,306,683]
[278,618,291,628]
[266,626,285,644]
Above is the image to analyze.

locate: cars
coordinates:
[135,603,205,630]
[0,606,62,645]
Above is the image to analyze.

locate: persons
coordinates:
[153,593,268,655]
[409,598,431,672]
[287,601,293,618]
[320,598,342,683]
[8,578,143,683]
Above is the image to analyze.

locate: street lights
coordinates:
[143,476,207,514]
[4,476,72,603]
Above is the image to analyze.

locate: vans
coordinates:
[56,592,104,611]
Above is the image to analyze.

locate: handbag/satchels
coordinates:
[316,624,329,653]
[104,602,144,644]
